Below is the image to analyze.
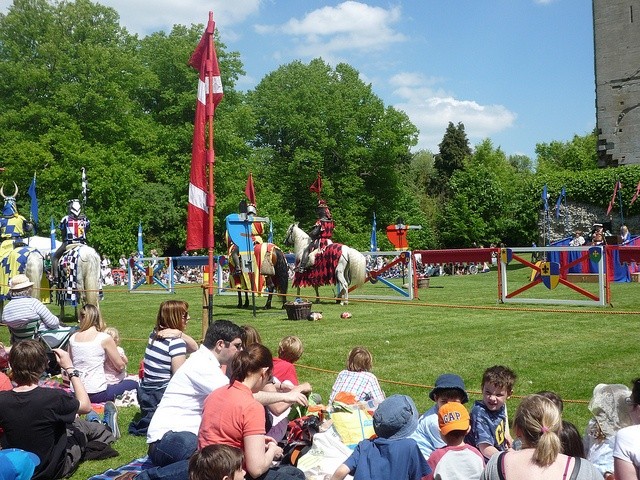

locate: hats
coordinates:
[318,204,329,218]
[1,180,18,214]
[247,201,256,216]
[67,200,81,216]
[438,401,471,436]
[587,383,633,436]
[373,394,419,441]
[8,274,35,290]
[428,374,468,404]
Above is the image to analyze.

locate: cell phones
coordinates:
[45,351,57,363]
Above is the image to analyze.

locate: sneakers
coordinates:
[85,408,101,425]
[112,471,138,480]
[100,401,120,440]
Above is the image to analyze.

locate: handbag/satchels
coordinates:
[331,392,381,448]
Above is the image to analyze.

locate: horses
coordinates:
[221,227,290,309]
[0,233,45,313]
[42,242,102,322]
[282,220,368,308]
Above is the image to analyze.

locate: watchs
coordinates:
[67,369,80,380]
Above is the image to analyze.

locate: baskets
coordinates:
[283,298,313,320]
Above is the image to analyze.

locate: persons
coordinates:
[0,180,33,260]
[619,226,631,245]
[585,382,632,480]
[530,241,539,262]
[195,343,307,480]
[112,318,309,480]
[230,203,264,276]
[465,365,517,464]
[422,401,486,480]
[569,230,586,246]
[522,390,584,458]
[480,394,605,480]
[590,222,606,234]
[100,249,230,288]
[365,239,504,280]
[329,345,385,403]
[66,303,139,403]
[0,338,123,479]
[128,299,199,435]
[293,203,335,273]
[612,375,640,479]
[187,444,248,480]
[0,273,80,348]
[50,197,90,282]
[587,233,606,247]
[104,326,128,385]
[0,341,14,393]
[409,373,469,459]
[226,324,313,443]
[270,335,303,386]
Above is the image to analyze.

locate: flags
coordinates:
[310,175,324,193]
[183,30,224,251]
[385,224,409,250]
[137,223,144,258]
[79,167,90,204]
[554,186,566,221]
[370,216,378,253]
[605,181,622,215]
[244,174,256,204]
[541,185,552,225]
[26,176,39,235]
[630,179,640,206]
[49,217,57,254]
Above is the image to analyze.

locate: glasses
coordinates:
[268,372,273,384]
[223,340,243,351]
[183,312,188,319]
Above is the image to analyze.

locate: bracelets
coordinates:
[624,241,626,244]
[290,402,294,408]
[64,366,76,371]
[279,380,285,391]
[177,331,183,338]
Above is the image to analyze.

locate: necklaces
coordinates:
[324,393,432,480]
